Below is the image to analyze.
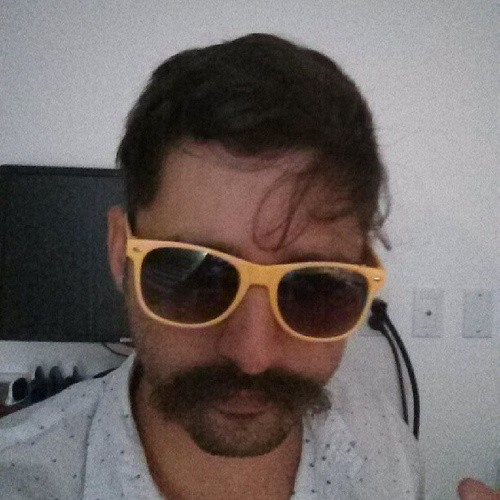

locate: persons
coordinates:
[0,32,500,500]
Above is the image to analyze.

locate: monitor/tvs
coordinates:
[0,164,138,347]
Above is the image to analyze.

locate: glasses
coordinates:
[121,210,387,343]
